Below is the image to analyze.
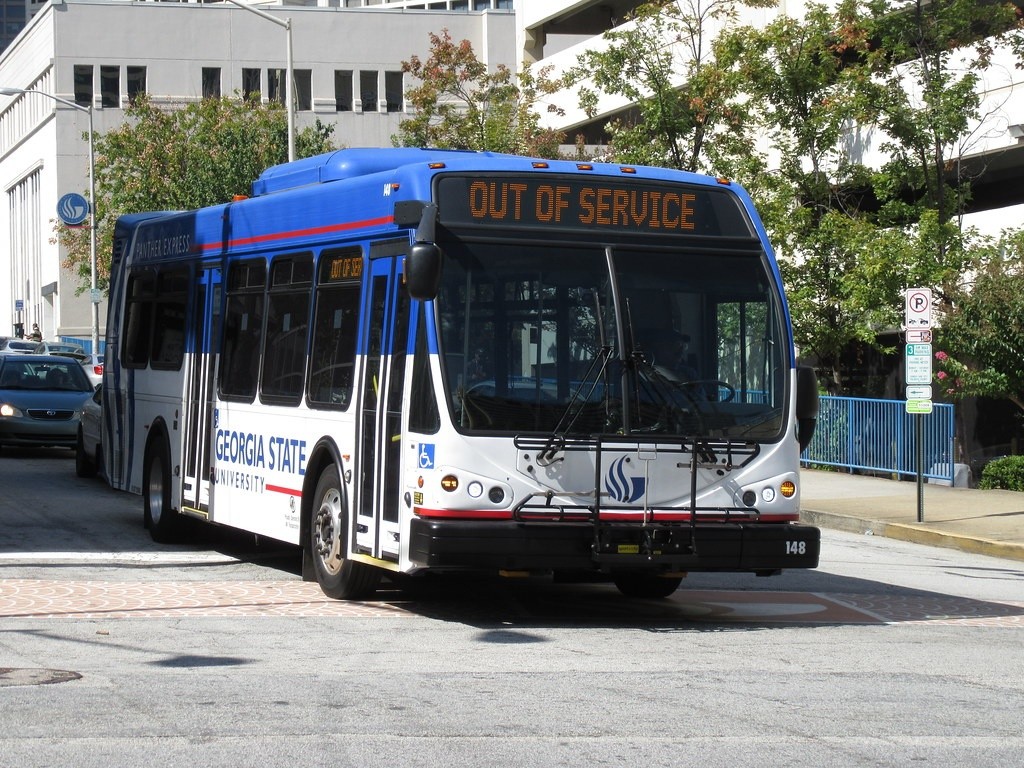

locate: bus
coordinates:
[103,143,823,602]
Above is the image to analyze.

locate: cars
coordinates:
[1,336,104,390]
[73,382,103,480]
[0,353,95,451]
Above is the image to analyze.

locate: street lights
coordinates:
[0,87,101,364]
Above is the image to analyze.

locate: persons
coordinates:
[47,369,80,390]
[650,329,708,402]
[25,323,42,342]
